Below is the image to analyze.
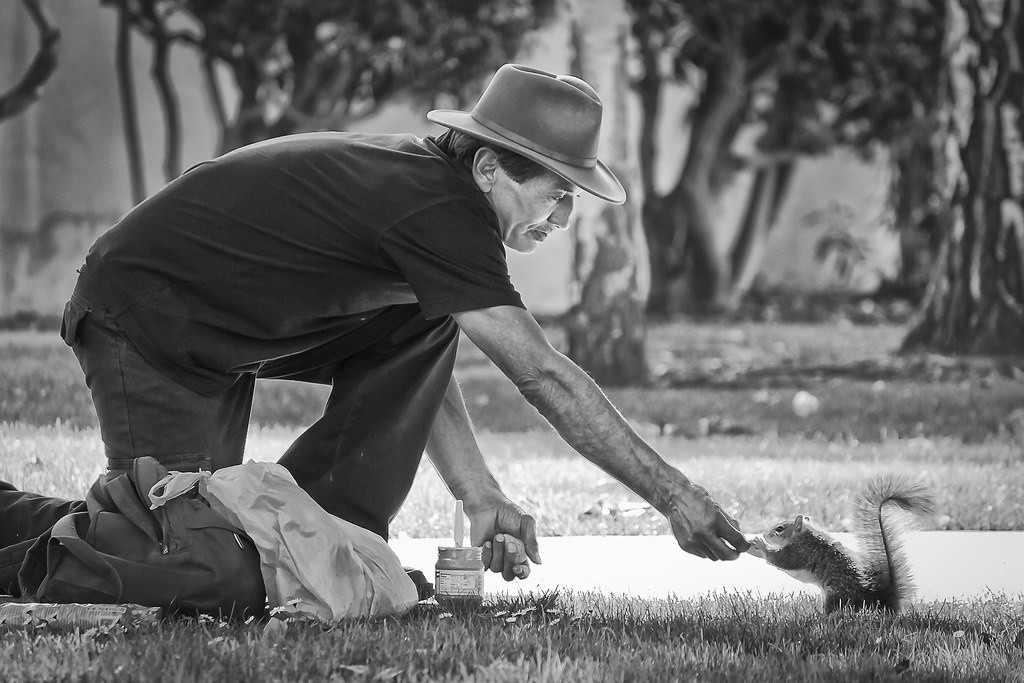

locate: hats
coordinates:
[426,63,627,206]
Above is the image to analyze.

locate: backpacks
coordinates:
[0,455,267,627]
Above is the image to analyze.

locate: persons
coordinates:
[0,64,750,602]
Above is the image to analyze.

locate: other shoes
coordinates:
[404,567,435,602]
